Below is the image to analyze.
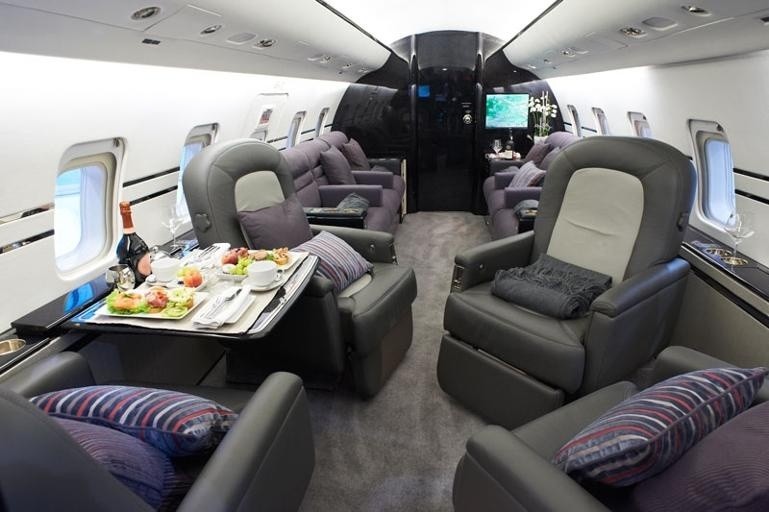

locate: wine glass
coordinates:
[493,139,503,159]
[162,212,185,250]
[721,211,756,265]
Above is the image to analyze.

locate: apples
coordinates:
[184,269,203,287]
[222,250,238,265]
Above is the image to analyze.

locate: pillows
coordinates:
[549,364,765,489]
[523,141,552,163]
[318,143,356,184]
[287,227,374,295]
[539,146,560,172]
[507,160,546,189]
[632,400,768,509]
[30,383,240,456]
[340,136,370,170]
[234,193,312,255]
[48,415,181,509]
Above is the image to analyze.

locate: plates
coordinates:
[240,276,288,293]
[94,290,257,325]
[216,249,297,280]
[142,272,210,291]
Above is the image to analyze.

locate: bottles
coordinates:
[115,203,152,282]
[503,127,516,161]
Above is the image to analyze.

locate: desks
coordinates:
[62,246,321,339]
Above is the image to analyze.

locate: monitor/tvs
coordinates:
[484,93,529,130]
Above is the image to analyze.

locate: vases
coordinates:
[534,134,548,146]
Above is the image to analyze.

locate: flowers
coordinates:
[527,94,559,135]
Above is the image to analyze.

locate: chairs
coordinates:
[277,145,392,236]
[491,186,541,240]
[481,130,581,222]
[318,131,405,223]
[2,345,319,512]
[434,136,698,428]
[293,138,402,220]
[450,344,768,509]
[180,135,418,401]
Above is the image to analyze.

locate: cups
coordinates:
[105,267,135,291]
[246,258,285,284]
[151,258,181,284]
